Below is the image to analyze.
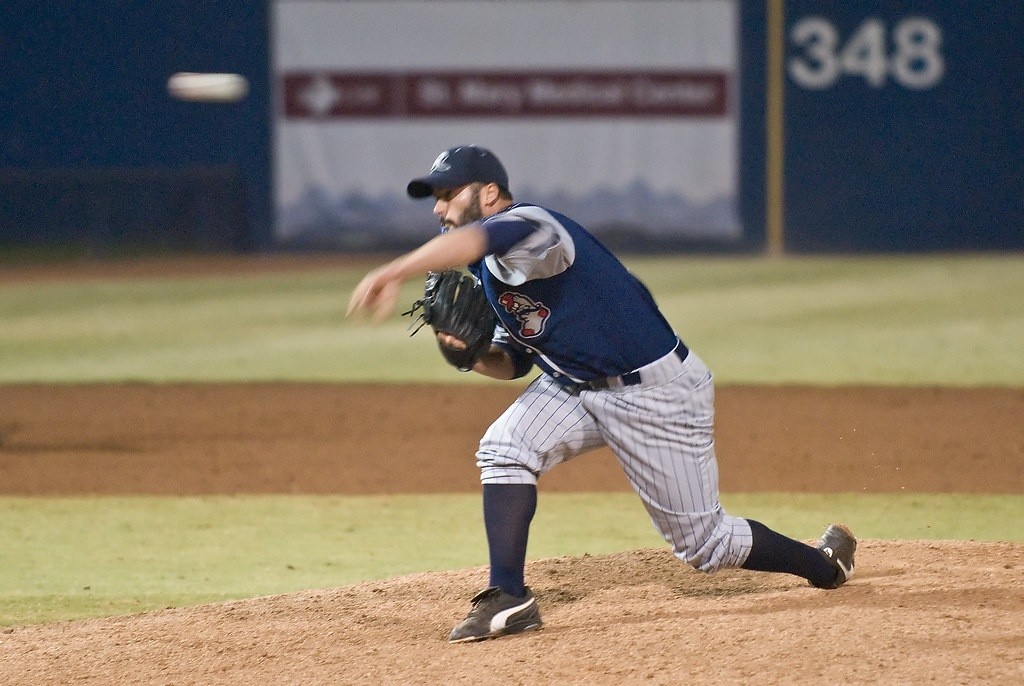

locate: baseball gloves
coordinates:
[401,268,495,372]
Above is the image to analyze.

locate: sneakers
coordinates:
[815,521,858,591]
[448,585,542,645]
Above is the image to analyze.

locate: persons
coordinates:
[345,145,857,643]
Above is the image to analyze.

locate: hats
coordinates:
[407,145,509,199]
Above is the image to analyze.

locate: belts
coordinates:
[589,339,688,392]
